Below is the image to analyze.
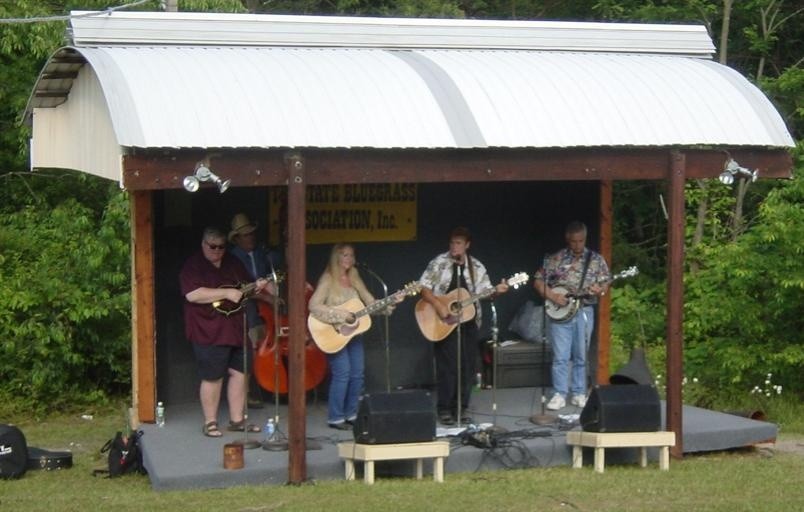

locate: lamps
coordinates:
[719,158,761,186]
[183,158,232,195]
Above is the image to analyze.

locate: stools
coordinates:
[565,431,675,473]
[336,442,450,485]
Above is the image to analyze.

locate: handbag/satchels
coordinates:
[92,430,152,485]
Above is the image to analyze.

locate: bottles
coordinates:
[156,402,165,428]
[266,418,275,440]
[476,367,492,389]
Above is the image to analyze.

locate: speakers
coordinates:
[353,389,436,444]
[580,385,661,433]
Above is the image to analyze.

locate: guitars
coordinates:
[213,270,286,316]
[307,281,421,354]
[415,272,529,341]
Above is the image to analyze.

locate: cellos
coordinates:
[254,229,327,393]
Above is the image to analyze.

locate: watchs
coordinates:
[600,288,605,297]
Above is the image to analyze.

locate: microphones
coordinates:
[258,242,271,256]
[350,259,369,272]
[453,253,465,266]
[542,250,550,265]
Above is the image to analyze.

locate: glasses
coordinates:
[204,241,225,249]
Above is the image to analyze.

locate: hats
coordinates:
[227,213,259,246]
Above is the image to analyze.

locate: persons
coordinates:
[179,225,269,439]
[534,220,609,411]
[224,214,284,410]
[418,226,508,425]
[307,243,396,430]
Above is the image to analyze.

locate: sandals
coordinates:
[227,419,259,432]
[202,421,222,437]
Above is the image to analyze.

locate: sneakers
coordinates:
[546,392,566,410]
[570,394,588,407]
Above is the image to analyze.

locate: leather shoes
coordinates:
[438,411,453,424]
[345,420,354,425]
[460,415,471,423]
[329,423,353,430]
